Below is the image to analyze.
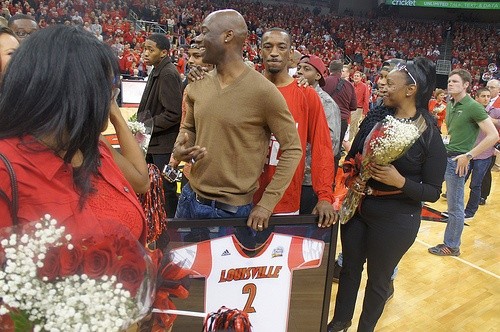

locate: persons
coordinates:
[327,56,447,332]
[172,10,303,232]
[428,69,500,257]
[0,23,150,332]
[105,100,150,193]
[0,0,500,110]
[184,28,405,304]
[136,32,184,219]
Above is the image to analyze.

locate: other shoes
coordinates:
[479,197,485,205]
[464,213,474,220]
[384,279,394,304]
[332,260,342,282]
[441,191,447,197]
[442,212,448,217]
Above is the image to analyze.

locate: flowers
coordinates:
[125,115,147,147]
[339,114,419,223]
[0,213,149,332]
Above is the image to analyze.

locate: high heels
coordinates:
[327,318,352,332]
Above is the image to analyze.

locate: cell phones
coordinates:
[163,165,180,182]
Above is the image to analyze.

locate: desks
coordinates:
[119,80,146,108]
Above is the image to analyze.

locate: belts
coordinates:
[366,187,403,195]
[188,181,239,213]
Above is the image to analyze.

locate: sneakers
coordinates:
[428,245,461,256]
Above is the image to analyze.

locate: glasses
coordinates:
[110,88,120,103]
[13,31,34,39]
[394,62,416,86]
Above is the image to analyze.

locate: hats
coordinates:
[297,54,328,87]
[380,59,404,72]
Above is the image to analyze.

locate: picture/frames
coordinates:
[141,214,339,332]
